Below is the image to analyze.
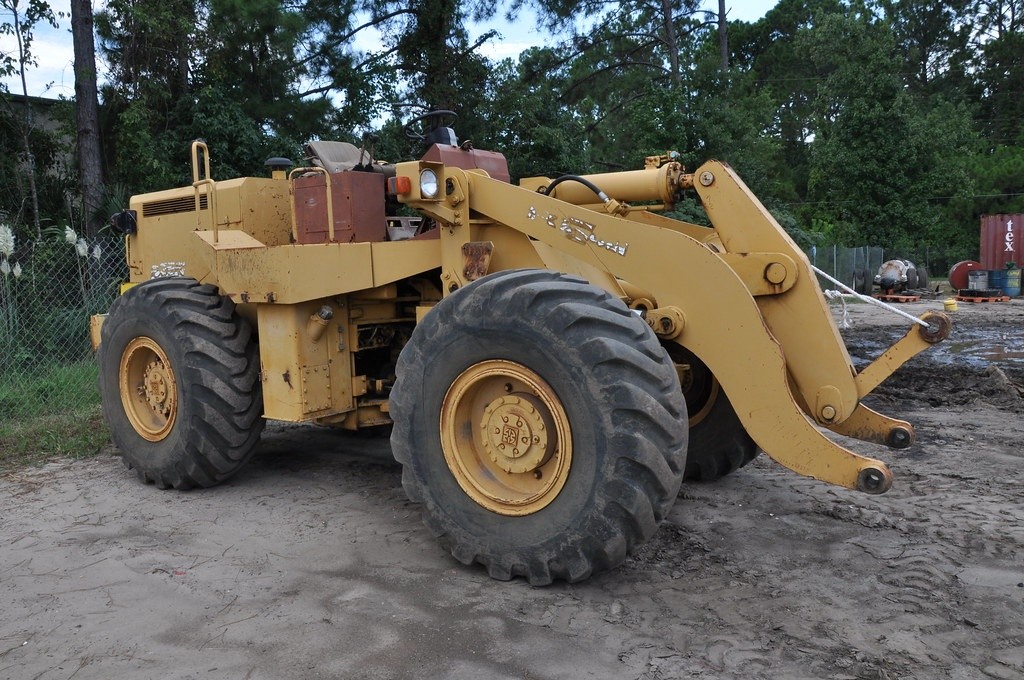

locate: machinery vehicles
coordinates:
[91,109,952,590]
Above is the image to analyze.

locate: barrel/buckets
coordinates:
[878,259,919,292]
[967,270,988,290]
[1001,268,1022,299]
[943,297,957,311]
[949,260,984,290]
[988,270,1002,289]
